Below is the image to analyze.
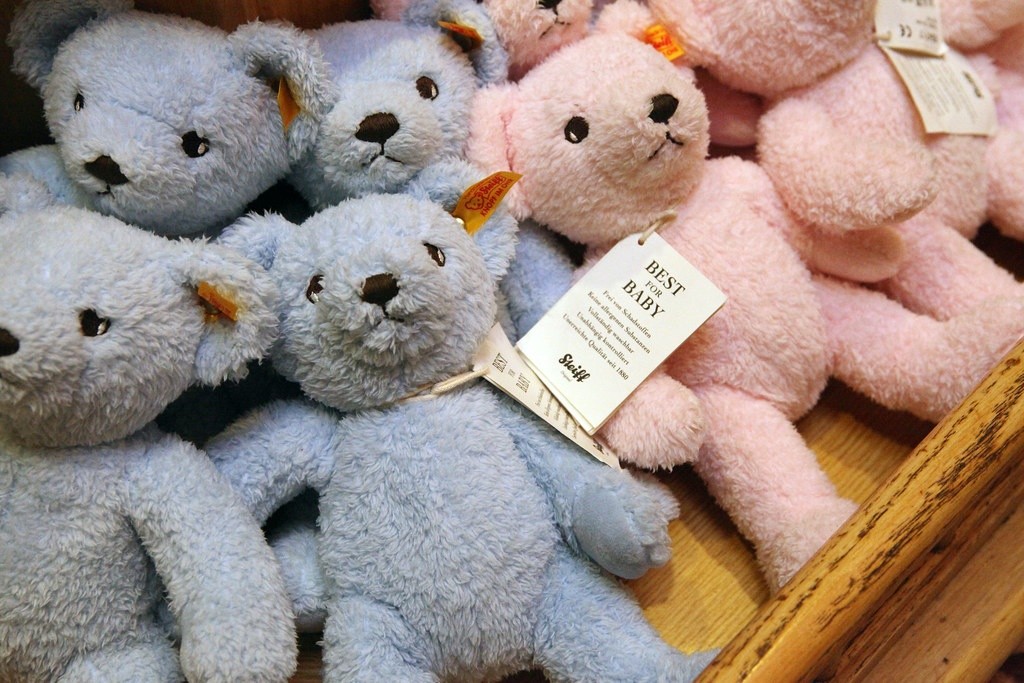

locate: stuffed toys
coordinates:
[469,1,1023,593]
[204,153,720,683]
[0,172,298,683]
[0,0,573,445]
